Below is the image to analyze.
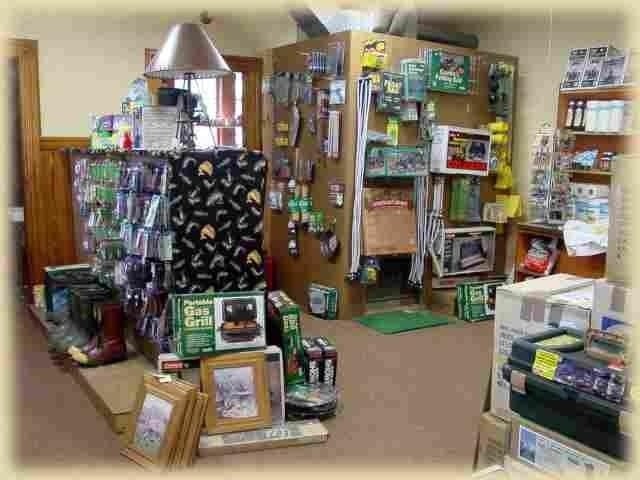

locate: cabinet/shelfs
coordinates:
[514,85,639,283]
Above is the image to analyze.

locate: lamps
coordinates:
[144,22,234,151]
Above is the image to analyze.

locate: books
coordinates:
[517,235,564,276]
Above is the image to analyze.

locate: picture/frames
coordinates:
[121,351,273,475]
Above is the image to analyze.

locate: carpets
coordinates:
[352,309,447,336]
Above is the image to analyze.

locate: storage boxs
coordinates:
[171,289,267,361]
[432,227,496,277]
[471,153,639,480]
[267,289,307,386]
[429,126,490,177]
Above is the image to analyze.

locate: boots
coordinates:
[47,283,128,368]
